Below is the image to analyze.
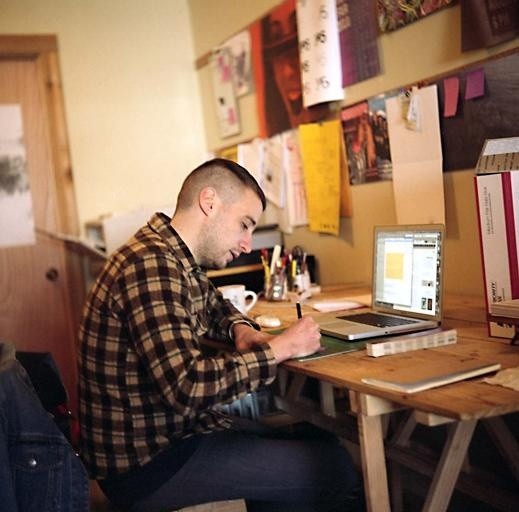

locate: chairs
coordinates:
[1,342,247,511]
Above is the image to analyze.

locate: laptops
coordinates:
[317,223,446,341]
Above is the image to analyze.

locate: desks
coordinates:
[245,283,518,512]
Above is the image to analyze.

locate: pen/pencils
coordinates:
[296,302,303,319]
[290,254,307,274]
[261,245,288,275]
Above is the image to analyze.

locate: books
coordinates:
[261,326,368,362]
[359,353,499,395]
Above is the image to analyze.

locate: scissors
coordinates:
[292,246,303,259]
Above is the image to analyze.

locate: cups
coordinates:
[264,275,288,301]
[215,285,257,317]
[290,272,311,299]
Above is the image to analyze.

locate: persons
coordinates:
[348,111,389,182]
[259,6,305,115]
[76,157,368,510]
